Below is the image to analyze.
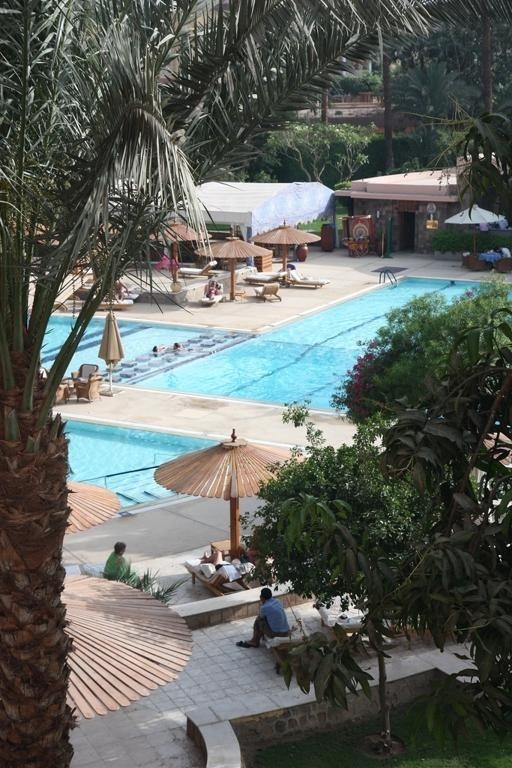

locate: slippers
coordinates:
[235,640,250,649]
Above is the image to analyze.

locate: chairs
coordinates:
[184,561,255,596]
[263,608,311,674]
[199,264,330,306]
[462,252,512,272]
[92,292,139,311]
[314,593,368,639]
[41,364,104,404]
[178,261,217,279]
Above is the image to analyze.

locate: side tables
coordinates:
[211,539,231,561]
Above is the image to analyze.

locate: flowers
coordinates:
[155,255,181,282]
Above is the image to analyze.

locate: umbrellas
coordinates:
[149,219,213,282]
[194,234,273,300]
[97,307,127,392]
[64,477,124,534]
[59,572,195,722]
[443,203,504,255]
[153,427,289,559]
[250,220,322,281]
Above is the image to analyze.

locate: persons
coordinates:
[153,345,170,353]
[173,342,216,355]
[243,587,291,647]
[200,549,243,572]
[103,542,132,582]
[206,278,219,301]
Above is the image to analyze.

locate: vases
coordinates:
[170,282,182,292]
